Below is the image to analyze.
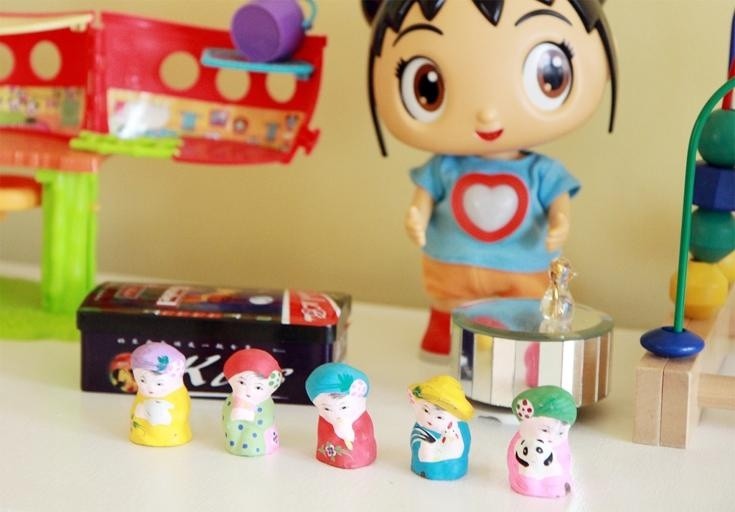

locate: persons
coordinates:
[128,339,194,447]
[306,362,377,469]
[507,384,580,499]
[407,374,475,481]
[220,347,286,457]
[359,0,619,363]
